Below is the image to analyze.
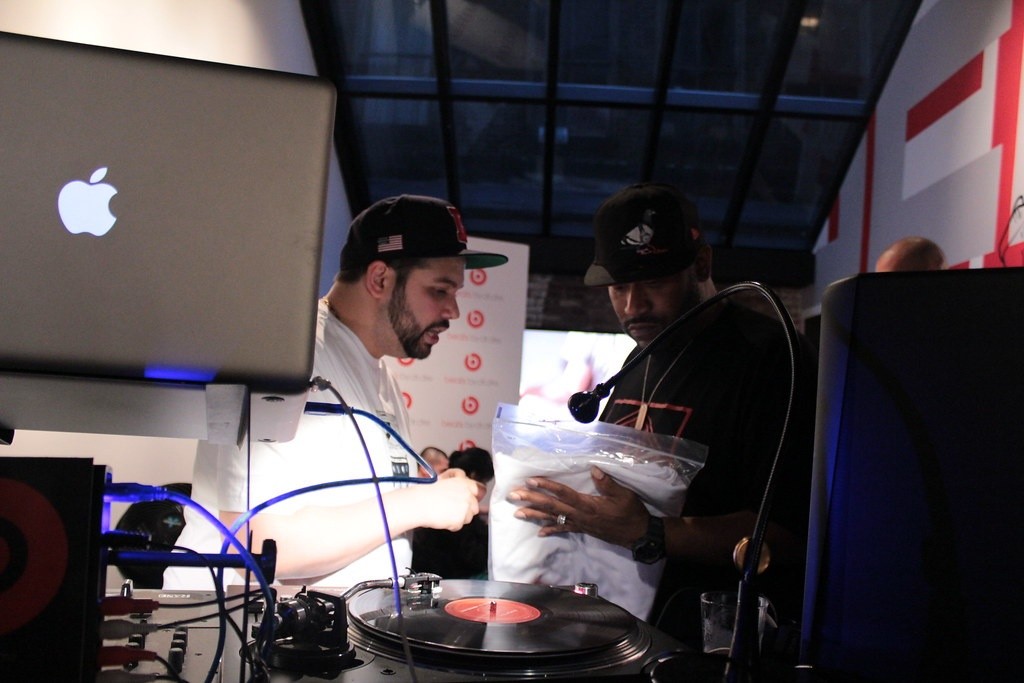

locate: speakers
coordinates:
[795,266,1024,683]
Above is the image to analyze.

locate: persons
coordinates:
[874,236,950,272]
[396,446,494,581]
[508,183,819,683]
[229,195,487,617]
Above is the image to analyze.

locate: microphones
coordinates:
[567,281,800,683]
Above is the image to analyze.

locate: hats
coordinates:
[584,184,705,287]
[341,193,508,269]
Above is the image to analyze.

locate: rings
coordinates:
[557,513,566,526]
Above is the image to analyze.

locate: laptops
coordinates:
[0,28,335,440]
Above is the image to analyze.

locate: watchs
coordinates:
[632,515,669,565]
